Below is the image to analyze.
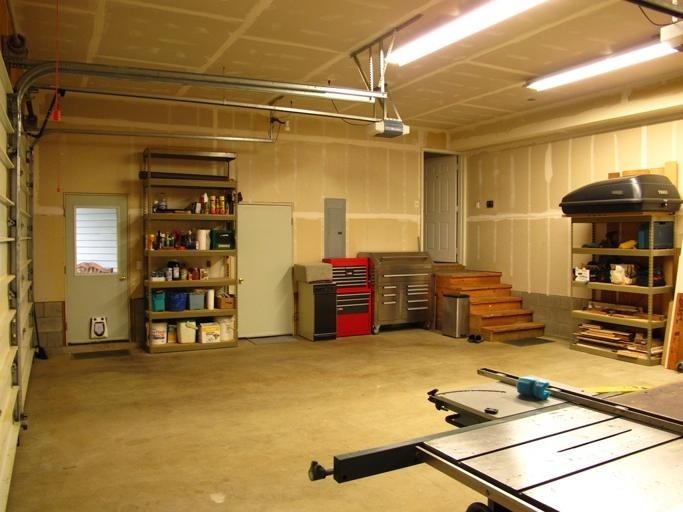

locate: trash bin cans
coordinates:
[441,294,470,339]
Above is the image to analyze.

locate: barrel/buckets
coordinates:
[145,321,169,346]
[167,291,188,311]
[176,319,198,344]
[196,228,213,251]
[212,316,236,342]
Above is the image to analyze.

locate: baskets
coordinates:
[216,294,235,308]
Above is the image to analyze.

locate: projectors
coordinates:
[368,122,410,138]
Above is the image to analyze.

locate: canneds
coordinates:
[167,259,180,281]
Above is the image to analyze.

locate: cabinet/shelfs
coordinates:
[564,211,678,365]
[0,56,32,512]
[137,143,241,353]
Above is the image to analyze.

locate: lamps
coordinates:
[383,0,541,66]
[523,42,679,93]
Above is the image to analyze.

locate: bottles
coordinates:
[153,192,226,281]
